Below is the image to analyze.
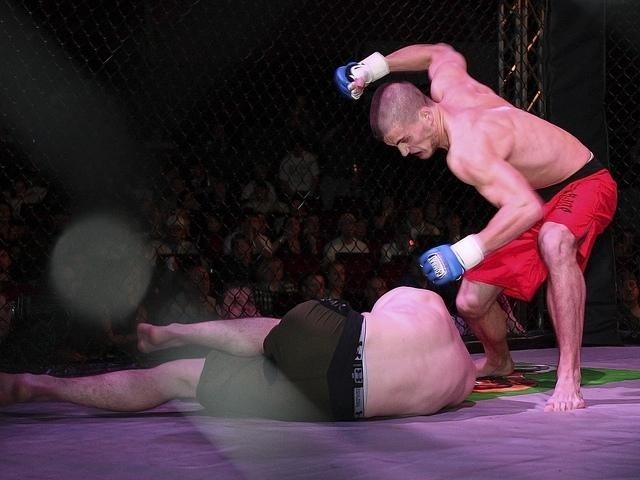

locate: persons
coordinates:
[332,40,618,411]
[2,285,477,423]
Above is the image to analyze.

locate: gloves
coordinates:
[417,234,484,286]
[334,51,390,101]
